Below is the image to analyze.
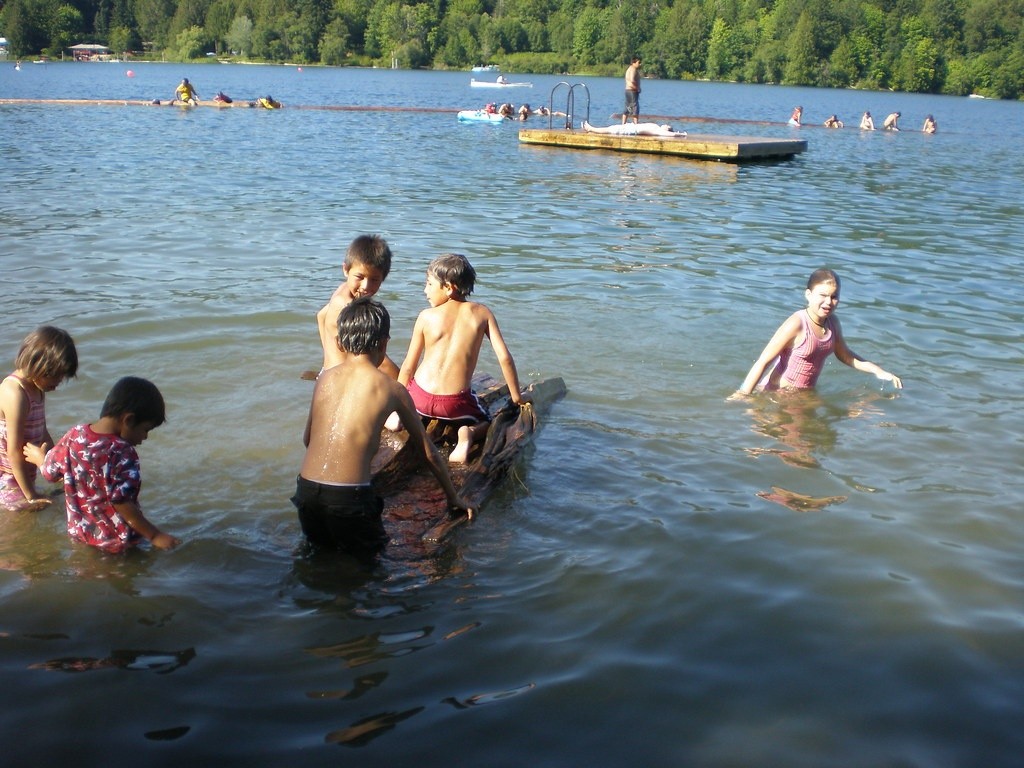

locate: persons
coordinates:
[823,115,845,129]
[724,268,904,403]
[303,234,403,453]
[1,325,82,514]
[175,78,200,107]
[24,376,183,551]
[213,91,234,108]
[788,106,802,128]
[883,112,902,132]
[480,102,573,124]
[579,120,689,139]
[620,56,641,126]
[922,114,937,133]
[393,249,535,463]
[248,95,284,110]
[289,297,476,555]
[859,111,876,131]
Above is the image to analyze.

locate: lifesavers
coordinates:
[458,110,505,122]
